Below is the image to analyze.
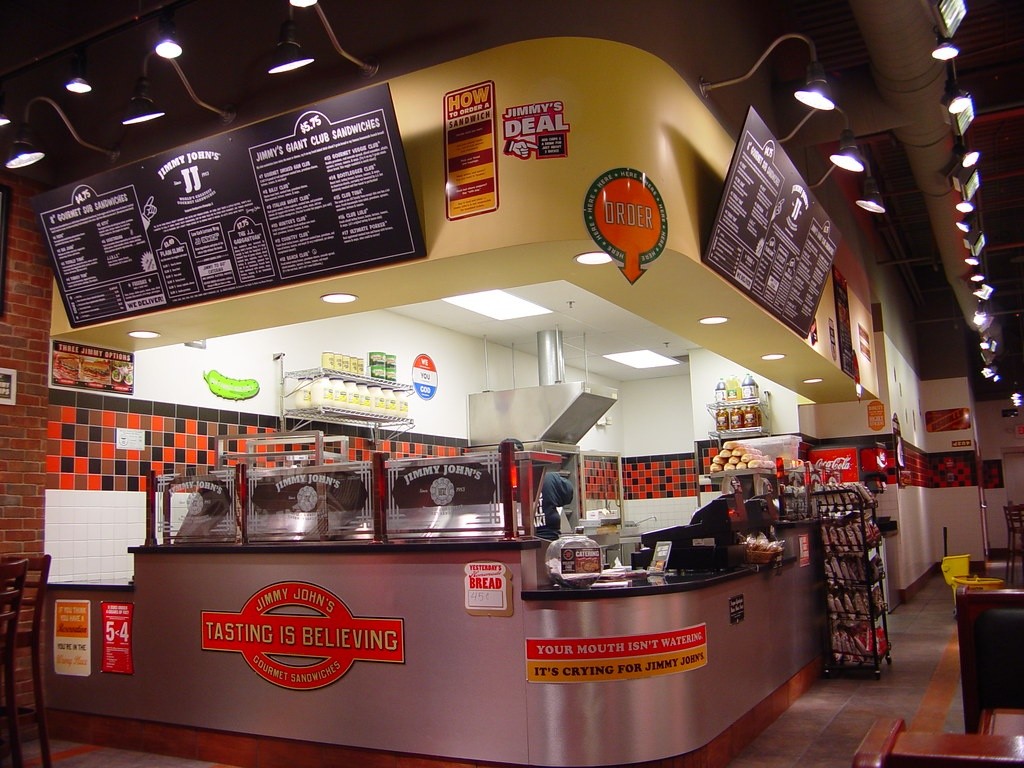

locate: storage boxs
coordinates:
[746,549,777,563]
[735,435,803,470]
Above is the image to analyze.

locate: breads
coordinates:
[744,537,780,552]
[710,441,761,472]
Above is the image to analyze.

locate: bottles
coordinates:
[543,533,604,591]
[283,375,408,418]
[715,373,759,405]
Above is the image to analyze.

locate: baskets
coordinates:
[746,546,786,563]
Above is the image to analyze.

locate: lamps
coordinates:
[969,281,995,301]
[265,0,380,81]
[807,154,887,215]
[119,51,238,128]
[928,0,968,39]
[774,106,866,173]
[0,112,12,127]
[941,99,975,137]
[152,11,183,61]
[951,166,980,201]
[962,231,986,257]
[956,212,974,234]
[924,32,960,61]
[953,143,982,169]
[696,33,836,112]
[63,50,93,95]
[971,300,1002,383]
[969,264,986,283]
[3,95,120,171]
[943,79,970,115]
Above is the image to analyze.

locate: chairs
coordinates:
[955,584,1024,735]
[848,706,1024,768]
[1002,505,1024,581]
[1007,504,1024,585]
[0,558,30,768]
[3,554,53,768]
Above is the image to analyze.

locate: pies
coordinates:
[111,361,134,385]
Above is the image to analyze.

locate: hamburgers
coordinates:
[82,362,109,380]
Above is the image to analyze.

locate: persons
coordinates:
[533,471,574,541]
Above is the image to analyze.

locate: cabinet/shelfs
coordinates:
[810,489,892,682]
[705,390,771,451]
[272,352,416,445]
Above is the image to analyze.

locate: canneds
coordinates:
[715,405,762,432]
[323,352,365,376]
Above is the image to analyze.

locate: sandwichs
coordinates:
[57,353,79,370]
